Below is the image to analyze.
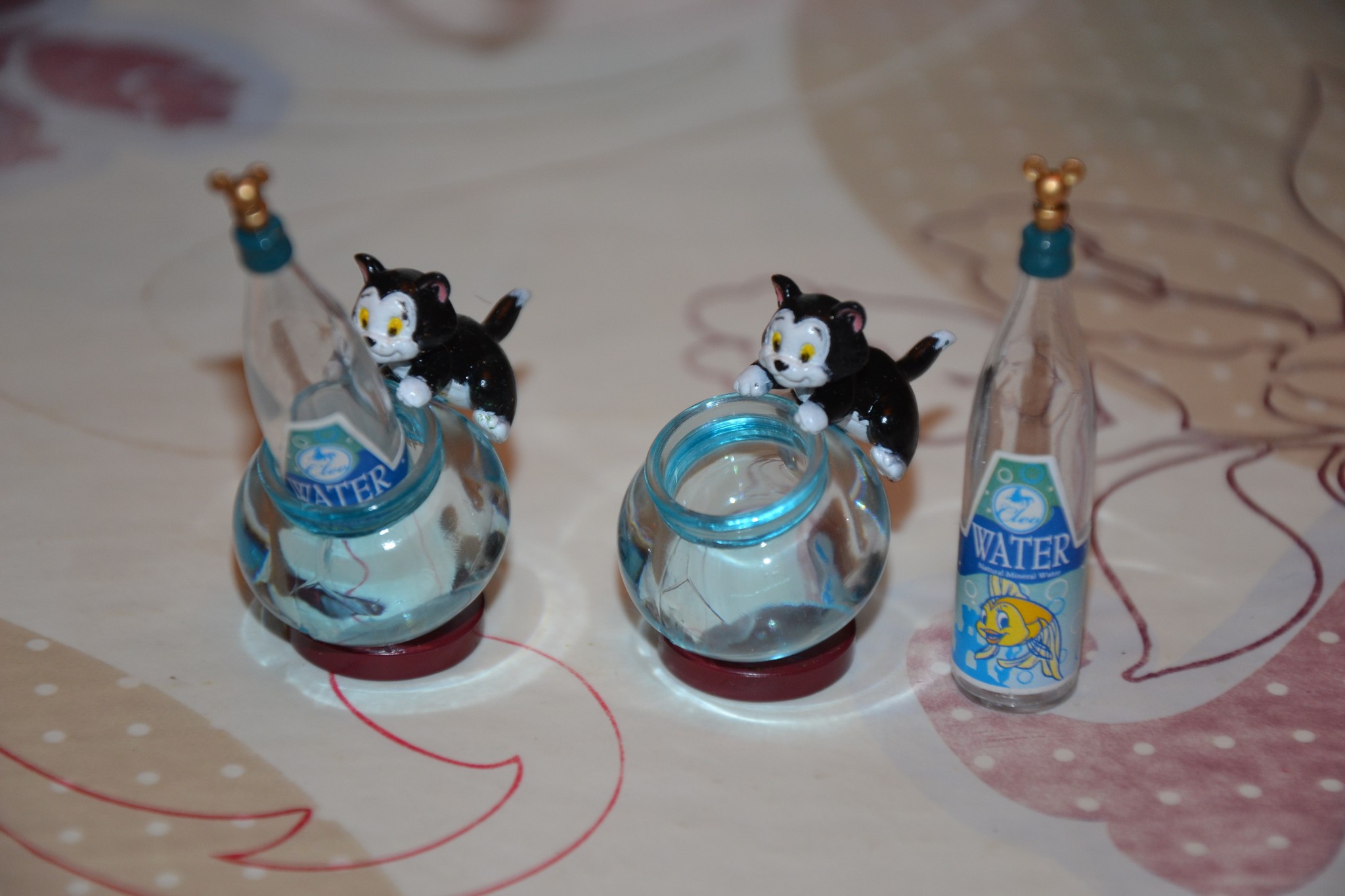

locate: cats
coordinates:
[732,274,957,483]
[349,253,531,442]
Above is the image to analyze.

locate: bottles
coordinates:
[212,162,415,511]
[949,213,1096,715]
